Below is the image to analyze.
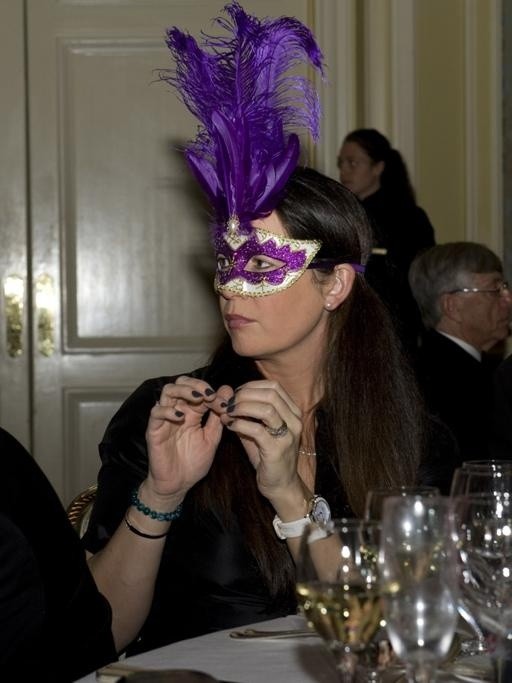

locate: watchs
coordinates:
[271,490,332,541]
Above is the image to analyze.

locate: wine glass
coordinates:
[294,458,512,683]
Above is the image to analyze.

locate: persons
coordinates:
[0,427,118,681]
[75,162,456,658]
[335,125,440,386]
[402,240,510,495]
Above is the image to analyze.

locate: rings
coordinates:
[264,421,290,437]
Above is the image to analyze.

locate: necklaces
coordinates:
[297,448,319,457]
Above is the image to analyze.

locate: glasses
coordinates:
[442,280,509,297]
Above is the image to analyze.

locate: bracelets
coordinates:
[124,510,170,540]
[129,485,184,522]
